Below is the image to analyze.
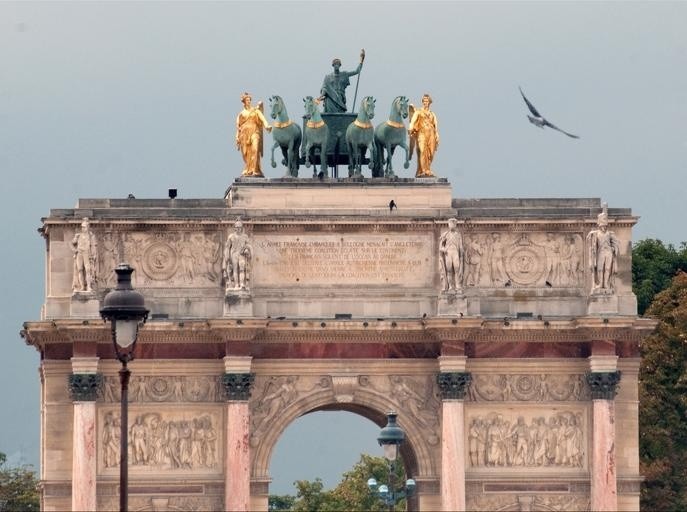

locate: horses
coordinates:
[374,95,410,179]
[302,95,328,180]
[345,96,376,177]
[268,94,302,178]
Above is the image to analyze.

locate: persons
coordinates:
[384,373,427,430]
[94,228,220,290]
[252,375,298,435]
[236,93,273,179]
[588,207,618,296]
[96,375,222,473]
[440,213,466,297]
[317,46,365,116]
[406,95,440,179]
[467,231,586,287]
[225,219,252,293]
[466,371,590,466]
[70,221,98,293]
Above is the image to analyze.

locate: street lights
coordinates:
[366,410,416,512]
[97,259,157,511]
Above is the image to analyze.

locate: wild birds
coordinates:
[518,85,581,140]
